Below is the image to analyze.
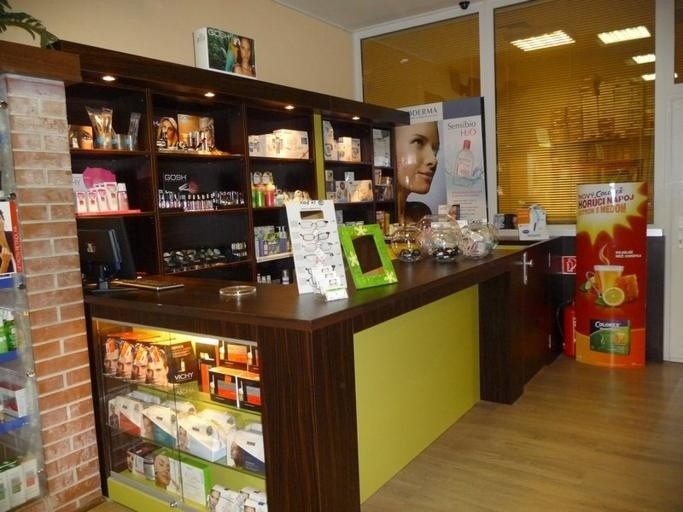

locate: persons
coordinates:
[199,117,214,148]
[395,121,440,229]
[210,489,220,511]
[0,210,16,274]
[230,441,245,471]
[103,337,180,390]
[143,415,154,443]
[79,126,94,150]
[157,116,179,147]
[109,404,119,429]
[232,35,256,78]
[244,506,256,512]
[154,455,171,492]
[176,427,190,453]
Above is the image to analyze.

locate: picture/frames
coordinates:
[337,225,398,289]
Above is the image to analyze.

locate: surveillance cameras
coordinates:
[459,1,470,9]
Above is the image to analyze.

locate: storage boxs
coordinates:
[550,77,655,140]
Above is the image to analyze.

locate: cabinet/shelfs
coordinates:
[0,72,48,512]
[46,38,410,285]
[90,316,269,512]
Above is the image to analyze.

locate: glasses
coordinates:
[296,219,335,287]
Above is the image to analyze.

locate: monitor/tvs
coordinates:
[76,216,136,292]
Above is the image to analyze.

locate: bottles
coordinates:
[453,140,476,188]
[256,269,289,286]
[0,307,21,353]
[256,226,289,257]
[392,213,501,262]
[186,194,214,210]
[252,184,275,208]
[188,130,210,152]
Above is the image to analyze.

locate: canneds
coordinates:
[253,189,274,207]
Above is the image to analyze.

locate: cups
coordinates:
[586,264,624,296]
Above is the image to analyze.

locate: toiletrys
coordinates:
[254,225,291,285]
[453,140,472,186]
[75,170,286,213]
[0,309,17,354]
[187,129,210,151]
